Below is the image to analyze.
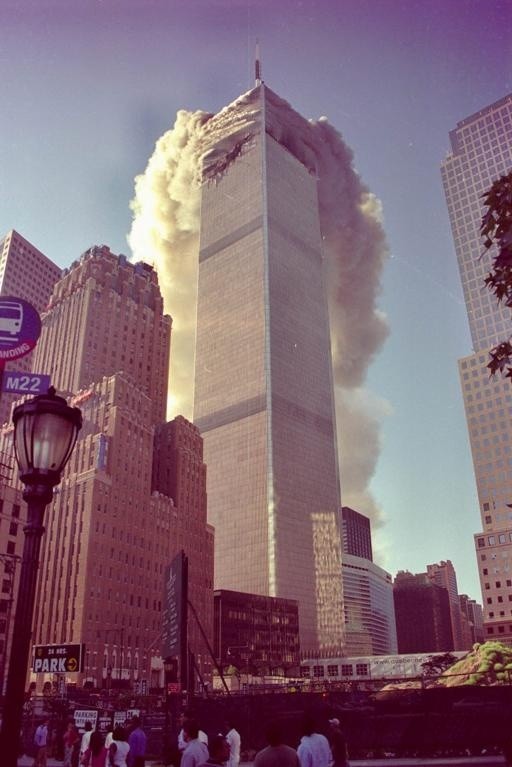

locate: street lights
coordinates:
[1,383,84,766]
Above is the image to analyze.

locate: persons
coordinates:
[33,716,347,767]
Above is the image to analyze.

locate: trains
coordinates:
[298,647,467,683]
